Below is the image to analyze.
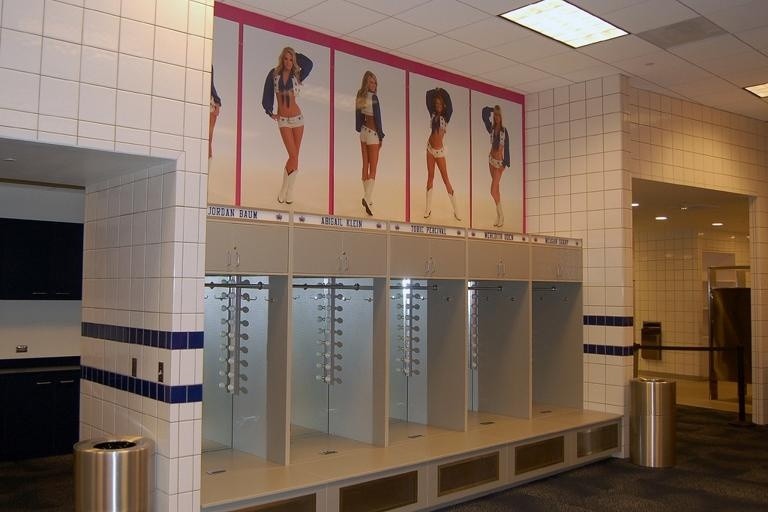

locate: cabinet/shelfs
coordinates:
[0,369,79,460]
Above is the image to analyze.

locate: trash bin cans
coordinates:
[629,376,676,468]
[72,434,155,512]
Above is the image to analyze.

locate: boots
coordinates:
[494,203,504,226]
[424,186,433,218]
[277,168,300,204]
[447,190,462,220]
[362,178,375,216]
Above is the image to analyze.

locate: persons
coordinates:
[206,63,222,203]
[261,46,313,204]
[424,86,463,221]
[354,70,384,216]
[481,105,512,228]
